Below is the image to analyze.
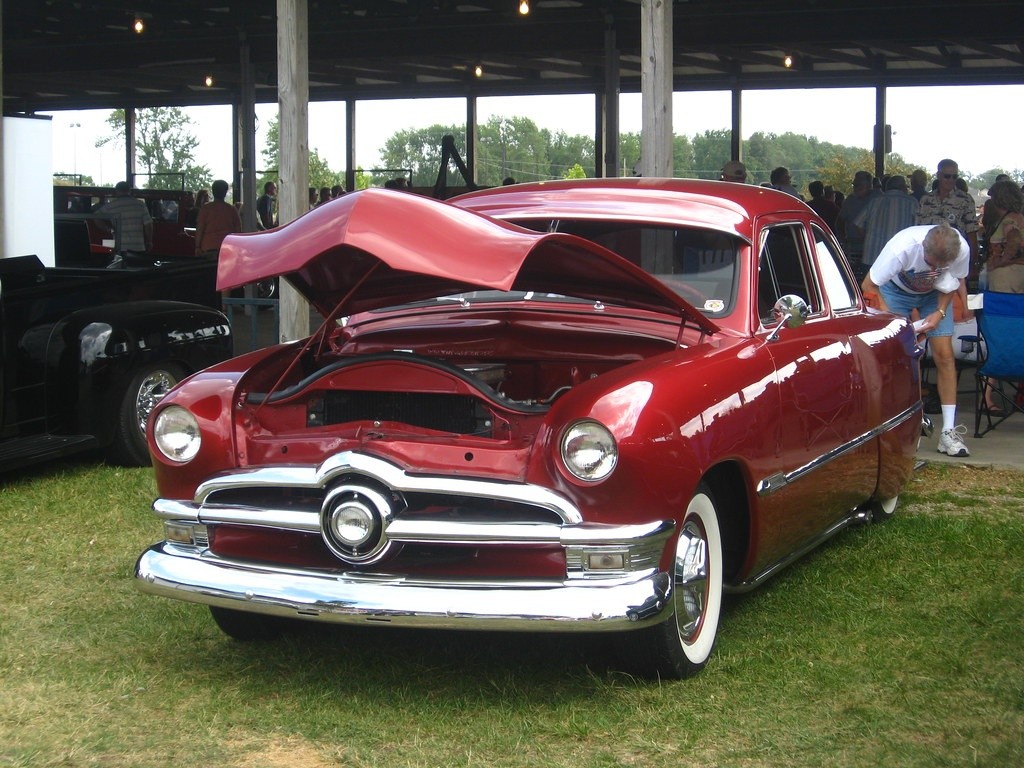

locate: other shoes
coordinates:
[976,401,1008,416]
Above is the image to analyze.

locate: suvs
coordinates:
[53,185,197,268]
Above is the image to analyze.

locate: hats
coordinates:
[907,170,928,180]
[113,181,131,191]
[723,161,748,178]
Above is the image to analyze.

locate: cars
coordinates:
[0,257,232,478]
[131,177,933,680]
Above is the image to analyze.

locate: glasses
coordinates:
[941,172,959,180]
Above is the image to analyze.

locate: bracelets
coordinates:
[937,308,947,321]
[195,246,201,249]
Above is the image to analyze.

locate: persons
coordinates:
[94,181,154,261]
[503,177,516,186]
[71,177,410,256]
[720,160,1024,417]
[862,225,970,456]
[194,180,242,317]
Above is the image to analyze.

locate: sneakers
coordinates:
[937,429,970,457]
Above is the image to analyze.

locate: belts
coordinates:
[201,249,217,253]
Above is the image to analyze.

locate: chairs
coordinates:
[673,231,773,318]
[921,289,1024,438]
